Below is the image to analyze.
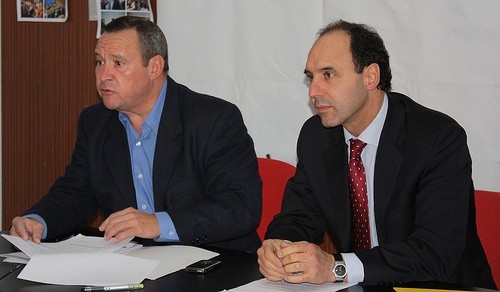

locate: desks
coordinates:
[0,226,490,292]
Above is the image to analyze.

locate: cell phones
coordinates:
[185,260,222,273]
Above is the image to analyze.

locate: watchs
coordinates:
[331,253,349,283]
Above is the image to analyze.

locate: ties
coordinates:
[347,138,371,255]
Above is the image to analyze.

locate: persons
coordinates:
[20,0,150,36]
[257,20,498,290]
[9,16,262,256]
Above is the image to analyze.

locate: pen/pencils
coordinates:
[80,283,145,292]
[55,230,77,241]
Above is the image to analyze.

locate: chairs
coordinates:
[473,190,500,292]
[256,157,296,245]
